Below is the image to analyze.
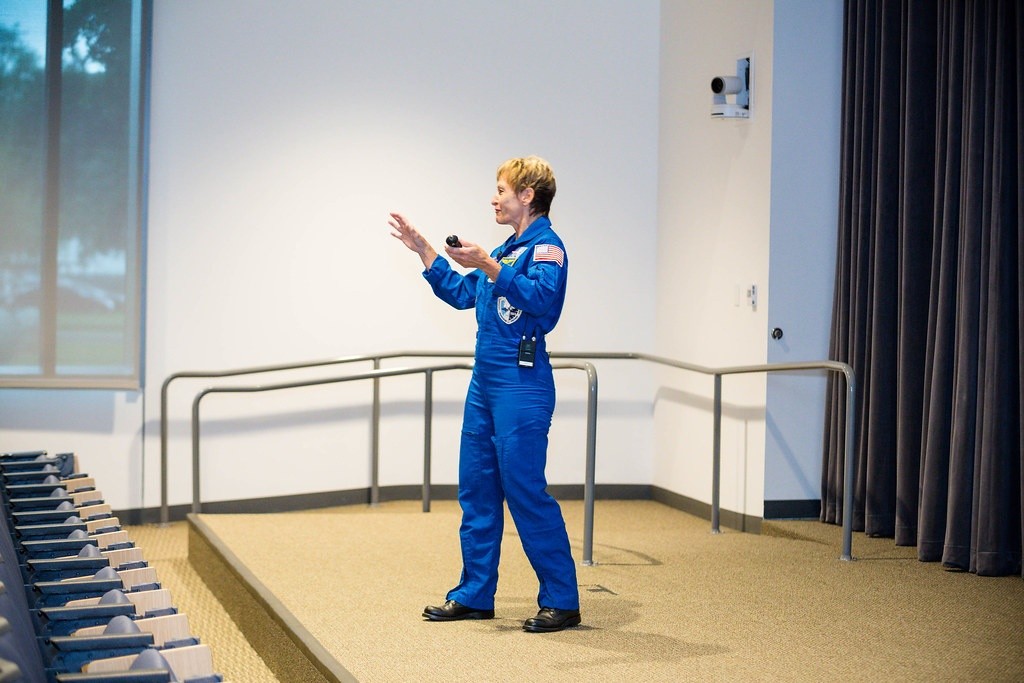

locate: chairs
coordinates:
[0,450,224,683]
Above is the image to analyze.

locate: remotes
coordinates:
[445,235,462,248]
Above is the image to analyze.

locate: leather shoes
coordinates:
[522,606,582,632]
[422,600,495,620]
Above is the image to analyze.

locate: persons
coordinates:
[388,155,582,633]
